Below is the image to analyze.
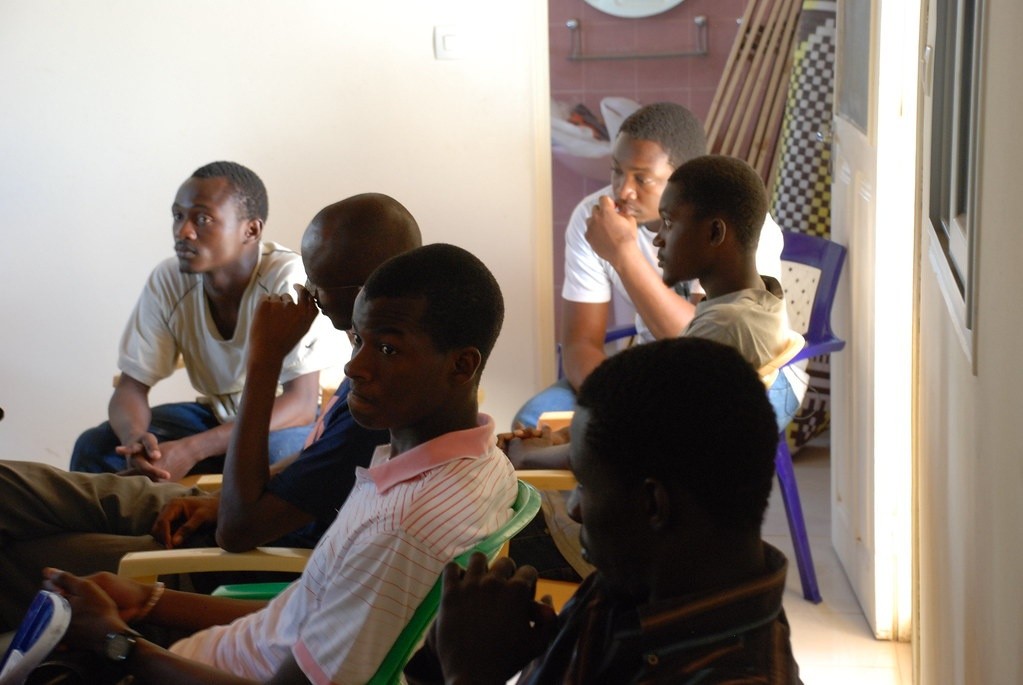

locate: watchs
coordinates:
[102,629,144,677]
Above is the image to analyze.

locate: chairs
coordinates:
[0,230,849,685]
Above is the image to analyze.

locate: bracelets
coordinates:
[137,582,164,621]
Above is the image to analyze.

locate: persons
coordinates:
[42,242,518,685]
[70,162,321,481]
[0,192,421,628]
[403,336,804,685]
[496,154,790,573]
[512,101,788,436]
